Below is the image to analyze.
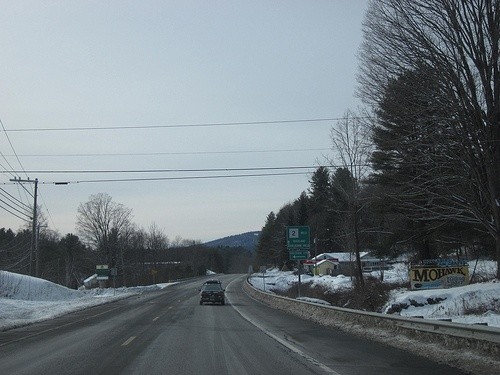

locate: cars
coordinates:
[198,283,225,305]
[203,280,222,287]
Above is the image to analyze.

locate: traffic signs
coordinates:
[286,226,310,250]
[289,251,308,260]
